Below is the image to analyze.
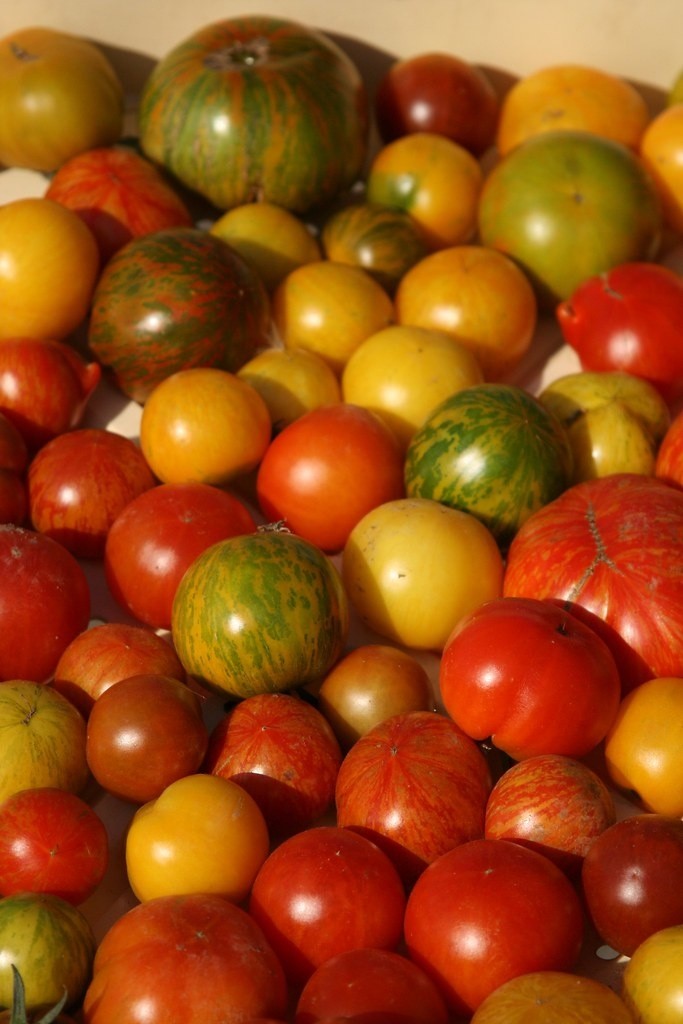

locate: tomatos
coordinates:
[0,13,683,1024]
